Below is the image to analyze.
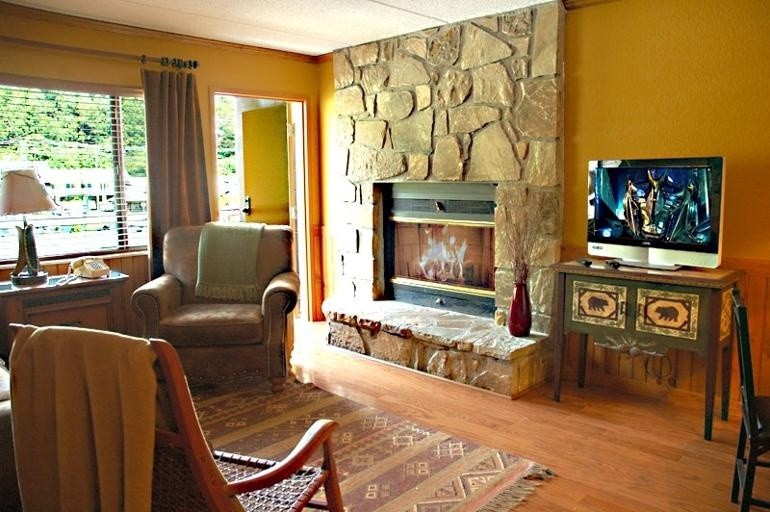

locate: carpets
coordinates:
[185,380,557,511]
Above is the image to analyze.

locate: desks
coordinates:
[0,269,130,366]
[550,260,746,442]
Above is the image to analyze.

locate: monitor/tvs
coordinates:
[587,156,726,271]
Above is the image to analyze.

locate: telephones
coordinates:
[67,255,111,281]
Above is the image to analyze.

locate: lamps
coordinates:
[0,168,61,286]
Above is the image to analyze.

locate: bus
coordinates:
[0,166,149,237]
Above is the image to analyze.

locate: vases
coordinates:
[508,279,532,338]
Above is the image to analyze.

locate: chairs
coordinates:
[129,223,302,381]
[4,322,345,510]
[728,282,770,511]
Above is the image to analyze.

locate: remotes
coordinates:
[576,258,592,266]
[606,260,620,269]
